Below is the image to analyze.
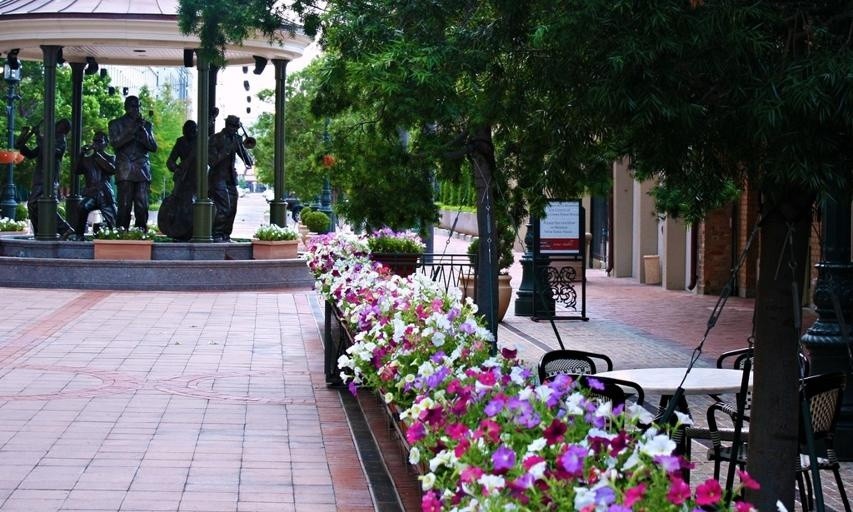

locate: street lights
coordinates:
[0,58,24,222]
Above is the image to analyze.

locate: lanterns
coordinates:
[319,156,334,168]
[13,151,24,164]
[0,148,18,163]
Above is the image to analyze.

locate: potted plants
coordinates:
[460,234,513,323]
[362,233,420,274]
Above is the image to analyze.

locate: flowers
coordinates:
[301,232,752,511]
[90,225,148,241]
[1,216,26,231]
[251,224,295,241]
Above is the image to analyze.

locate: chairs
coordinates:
[535,342,646,410]
[683,345,852,512]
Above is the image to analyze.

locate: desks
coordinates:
[594,368,756,500]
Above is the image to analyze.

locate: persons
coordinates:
[72,130,117,240]
[107,95,158,235]
[165,119,199,205]
[14,118,75,241]
[208,113,252,243]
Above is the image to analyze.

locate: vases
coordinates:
[93,239,155,261]
[251,239,299,257]
